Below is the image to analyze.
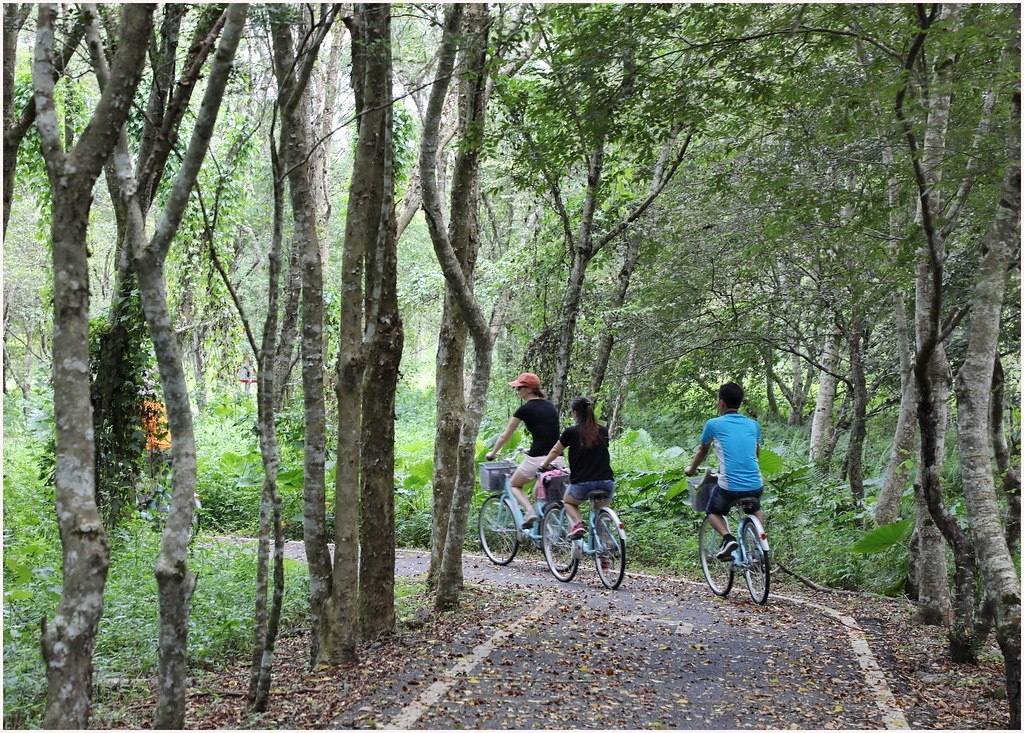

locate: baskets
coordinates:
[687,476,718,512]
[536,473,569,501]
[478,461,517,492]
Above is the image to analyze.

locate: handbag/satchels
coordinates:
[694,467,720,496]
[537,470,566,499]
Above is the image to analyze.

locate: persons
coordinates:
[685,382,771,574]
[540,397,614,570]
[484,373,565,530]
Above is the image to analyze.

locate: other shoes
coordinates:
[524,529,542,548]
[522,509,538,529]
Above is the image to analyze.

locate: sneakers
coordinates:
[600,557,609,571]
[567,522,587,538]
[716,536,738,559]
[749,561,766,575]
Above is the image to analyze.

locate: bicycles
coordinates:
[534,462,627,591]
[477,446,574,574]
[686,466,771,606]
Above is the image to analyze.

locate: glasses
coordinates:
[516,386,528,391]
[570,411,575,415]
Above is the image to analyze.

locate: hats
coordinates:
[508,373,540,389]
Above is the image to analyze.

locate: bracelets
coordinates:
[544,462,549,467]
